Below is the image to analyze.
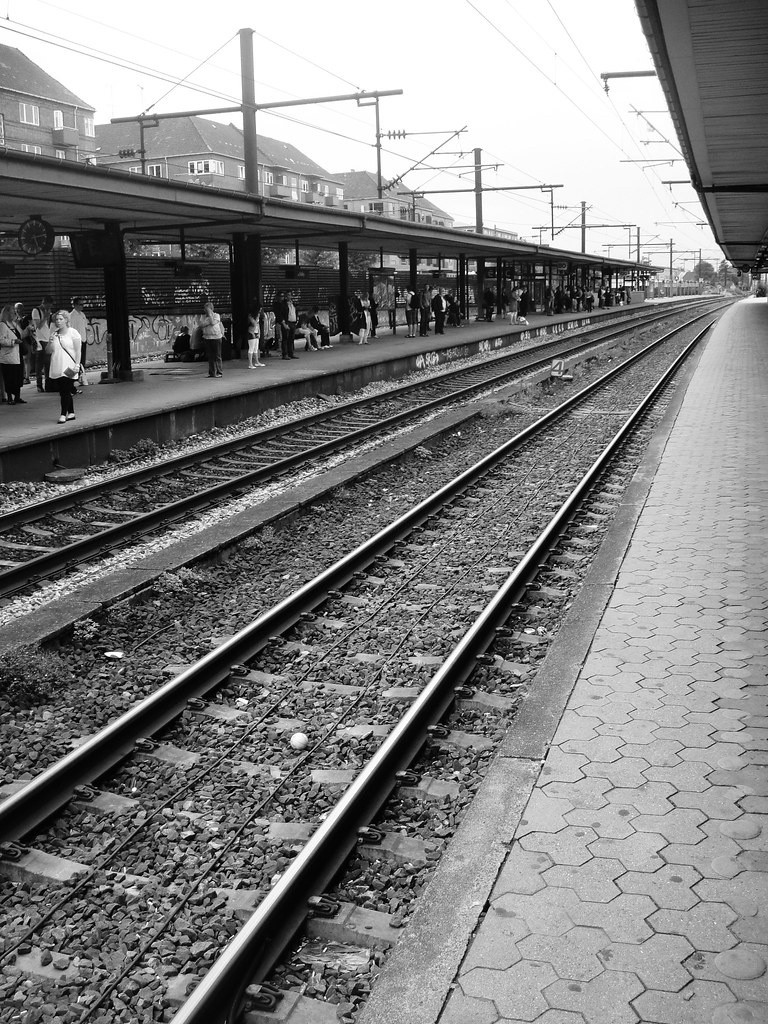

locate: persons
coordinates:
[357,292,380,345]
[402,283,463,338]
[484,288,495,322]
[0,296,88,423]
[598,284,635,308]
[247,304,265,369]
[273,290,299,360]
[502,286,529,325]
[173,303,223,378]
[297,306,333,351]
[546,284,595,315]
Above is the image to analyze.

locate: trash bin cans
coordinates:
[221,318,231,351]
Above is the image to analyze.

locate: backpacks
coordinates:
[408,293,420,309]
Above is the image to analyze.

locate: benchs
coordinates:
[295,332,321,339]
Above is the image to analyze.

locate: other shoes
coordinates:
[14,398,27,404]
[282,355,291,359]
[289,356,299,359]
[255,362,265,367]
[372,335,379,339]
[37,385,44,392]
[405,333,415,338]
[58,413,66,423]
[249,365,257,369]
[364,342,369,344]
[328,344,334,347]
[420,333,428,337]
[68,413,75,420]
[322,344,329,349]
[316,345,324,350]
[215,374,222,378]
[7,398,14,404]
[358,341,362,345]
[308,345,317,351]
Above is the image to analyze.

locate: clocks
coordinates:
[17,219,55,256]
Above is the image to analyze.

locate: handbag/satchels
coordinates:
[29,330,42,354]
[78,364,88,386]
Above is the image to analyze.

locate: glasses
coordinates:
[76,303,83,306]
